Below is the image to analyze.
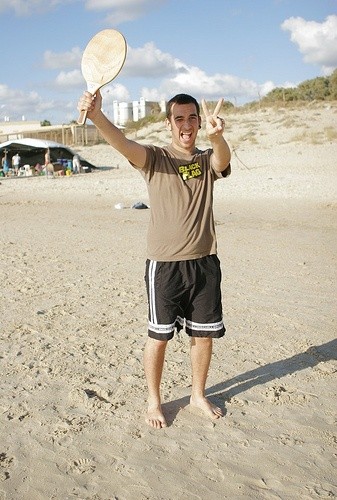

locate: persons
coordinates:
[72,154,81,175]
[75,89,233,428]
[1,155,9,178]
[44,149,54,179]
[11,153,21,176]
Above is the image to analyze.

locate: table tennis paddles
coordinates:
[77,28,126,125]
